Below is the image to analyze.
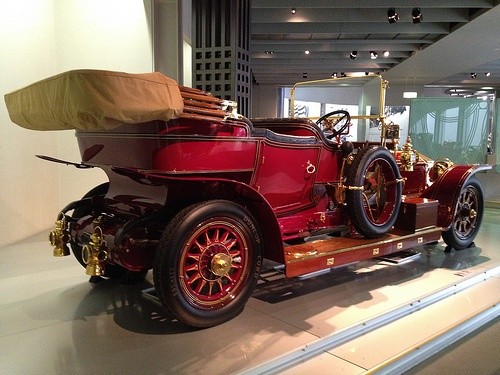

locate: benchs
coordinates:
[224,116,340,210]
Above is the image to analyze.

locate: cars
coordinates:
[4,75,493,329]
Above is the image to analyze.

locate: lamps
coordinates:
[331,73,338,79]
[470,72,477,79]
[387,7,400,24]
[349,51,357,60]
[291,8,297,16]
[411,7,424,25]
[340,72,347,78]
[370,51,378,59]
